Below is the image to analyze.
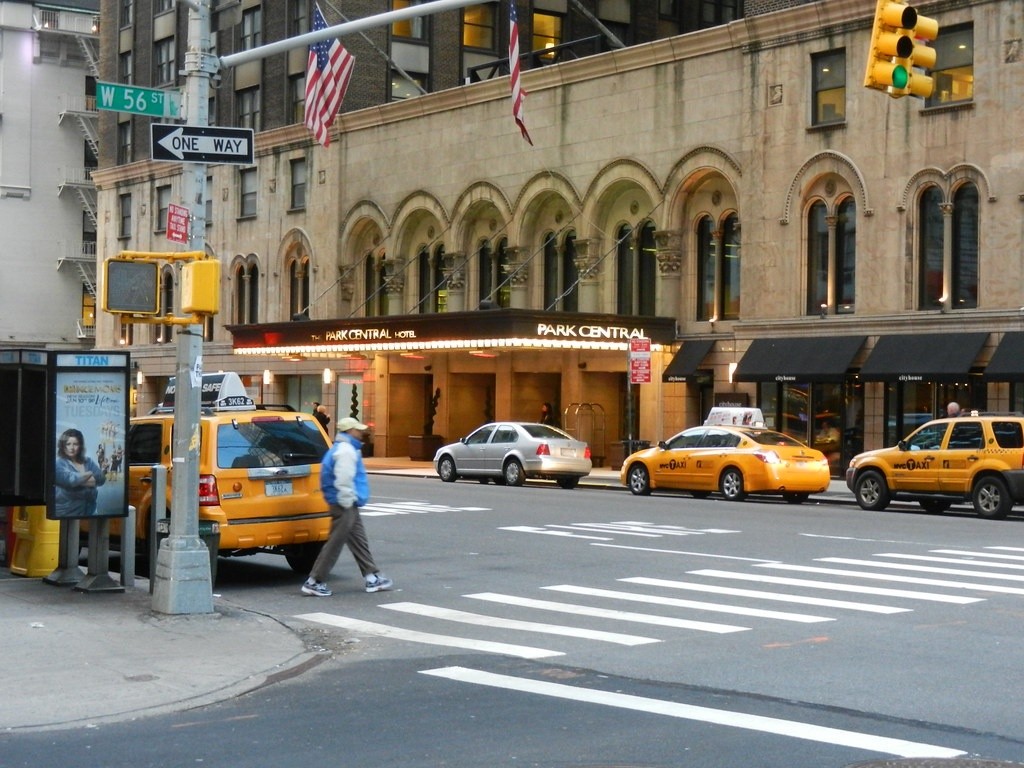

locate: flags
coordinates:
[509,0,534,146]
[302,0,356,149]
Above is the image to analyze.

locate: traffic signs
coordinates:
[96,81,181,122]
[152,123,256,167]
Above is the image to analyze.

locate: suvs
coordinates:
[80,371,334,575]
[846,411,1024,520]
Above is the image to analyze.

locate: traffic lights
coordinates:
[892,10,937,97]
[863,0,917,95]
[104,258,160,315]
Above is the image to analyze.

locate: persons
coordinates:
[315,405,330,437]
[300,417,394,596]
[312,402,321,416]
[541,402,553,426]
[96,444,124,481]
[55,429,107,516]
[944,401,961,431]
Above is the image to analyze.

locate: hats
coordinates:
[337,417,368,432]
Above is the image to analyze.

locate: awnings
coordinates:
[662,340,718,383]
[732,331,1024,383]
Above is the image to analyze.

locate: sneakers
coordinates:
[366,575,394,593]
[301,580,333,596]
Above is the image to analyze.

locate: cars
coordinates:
[432,422,592,489]
[621,407,831,503]
